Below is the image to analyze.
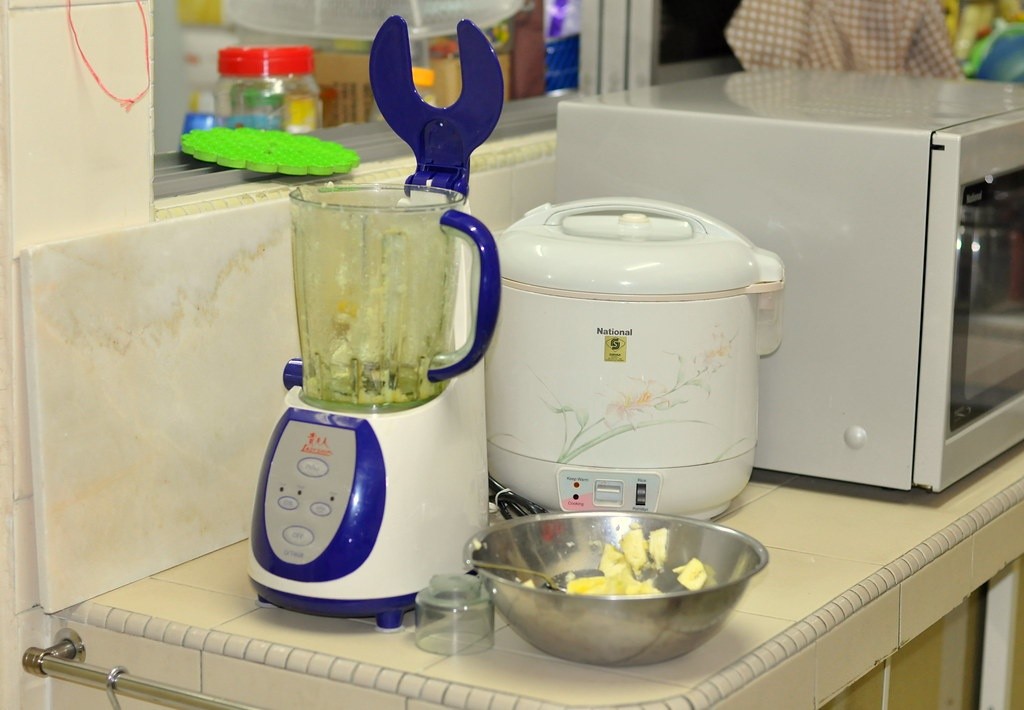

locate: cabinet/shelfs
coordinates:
[73,447,1024,709]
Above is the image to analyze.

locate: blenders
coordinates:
[241,16,511,628]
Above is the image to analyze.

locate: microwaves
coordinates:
[552,66,1024,498]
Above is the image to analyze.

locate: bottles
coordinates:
[415,576,499,657]
[211,43,323,140]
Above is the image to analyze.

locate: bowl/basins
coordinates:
[465,511,769,668]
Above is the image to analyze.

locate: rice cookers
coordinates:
[476,195,794,524]
